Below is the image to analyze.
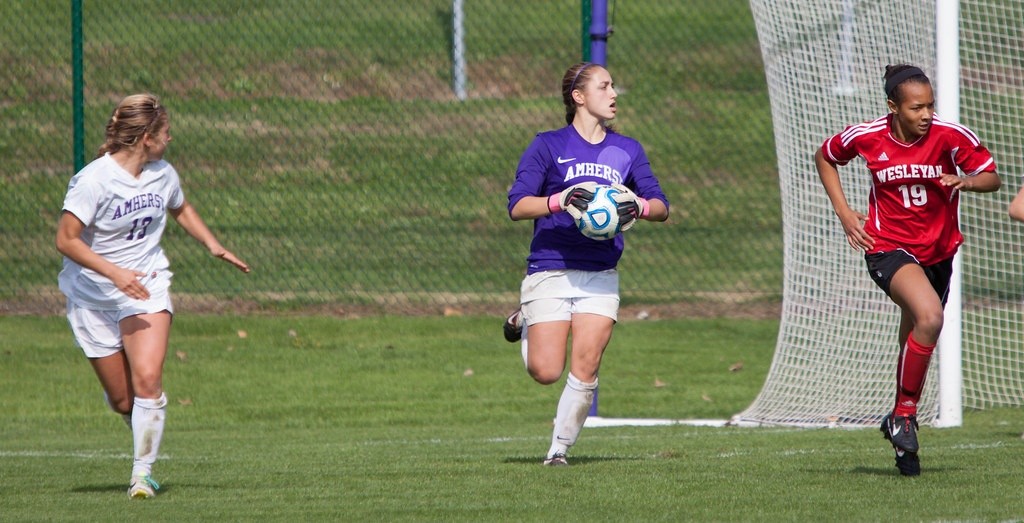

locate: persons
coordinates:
[503,61,670,465]
[814,65,1002,474]
[1009,187,1024,221]
[55,92,251,496]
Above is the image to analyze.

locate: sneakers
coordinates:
[503,307,522,343]
[126,475,159,500]
[543,450,568,466]
[120,414,131,430]
[879,413,921,476]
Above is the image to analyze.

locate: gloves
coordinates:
[611,181,650,232]
[546,181,598,220]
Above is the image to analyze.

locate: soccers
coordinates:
[574,184,626,242]
